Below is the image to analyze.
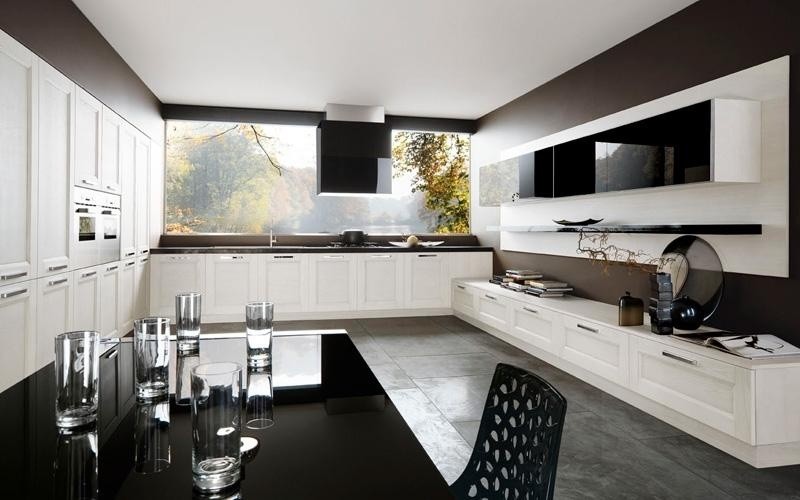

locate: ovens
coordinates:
[74,186,121,269]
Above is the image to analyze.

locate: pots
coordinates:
[339,230,368,247]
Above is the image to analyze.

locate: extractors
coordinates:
[316,120,392,198]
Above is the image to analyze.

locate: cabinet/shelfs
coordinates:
[1,30,152,393]
[450,275,797,469]
[515,97,764,237]
[151,231,494,323]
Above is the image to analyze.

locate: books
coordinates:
[670,324,800,358]
[490,268,575,298]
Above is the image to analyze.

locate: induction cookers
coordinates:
[329,240,378,248]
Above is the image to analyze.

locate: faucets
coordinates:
[269,227,277,247]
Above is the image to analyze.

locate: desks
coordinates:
[0,334,455,500]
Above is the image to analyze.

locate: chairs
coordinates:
[449,362,568,500]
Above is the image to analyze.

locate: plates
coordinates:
[387,240,445,247]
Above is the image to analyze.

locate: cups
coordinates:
[133,318,170,396]
[176,292,201,348]
[54,330,100,427]
[246,302,273,360]
[52,427,98,499]
[246,361,274,430]
[191,361,242,490]
[175,347,201,405]
[132,397,169,476]
